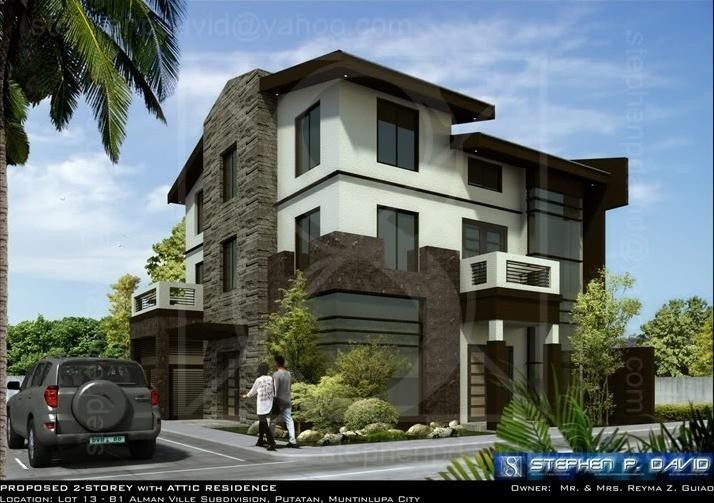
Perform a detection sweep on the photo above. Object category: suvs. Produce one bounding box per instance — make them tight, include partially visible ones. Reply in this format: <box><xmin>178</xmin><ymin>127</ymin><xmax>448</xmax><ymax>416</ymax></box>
<box><xmin>7</xmin><ymin>357</ymin><xmax>162</xmax><ymax>468</ymax></box>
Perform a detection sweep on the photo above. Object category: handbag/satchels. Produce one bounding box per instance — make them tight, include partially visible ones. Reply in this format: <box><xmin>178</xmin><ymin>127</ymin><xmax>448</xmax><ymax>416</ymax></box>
<box><xmin>270</xmin><ymin>396</ymin><xmax>281</xmax><ymax>416</ymax></box>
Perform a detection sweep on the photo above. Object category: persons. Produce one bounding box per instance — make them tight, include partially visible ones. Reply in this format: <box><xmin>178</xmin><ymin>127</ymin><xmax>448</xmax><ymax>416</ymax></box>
<box><xmin>240</xmin><ymin>360</ymin><xmax>277</xmax><ymax>450</ymax></box>
<box><xmin>268</xmin><ymin>355</ymin><xmax>298</xmax><ymax>447</ymax></box>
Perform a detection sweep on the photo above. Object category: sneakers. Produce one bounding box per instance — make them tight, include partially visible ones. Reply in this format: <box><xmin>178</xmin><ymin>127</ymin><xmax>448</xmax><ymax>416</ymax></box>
<box><xmin>253</xmin><ymin>439</ymin><xmax>298</xmax><ymax>451</ymax></box>
